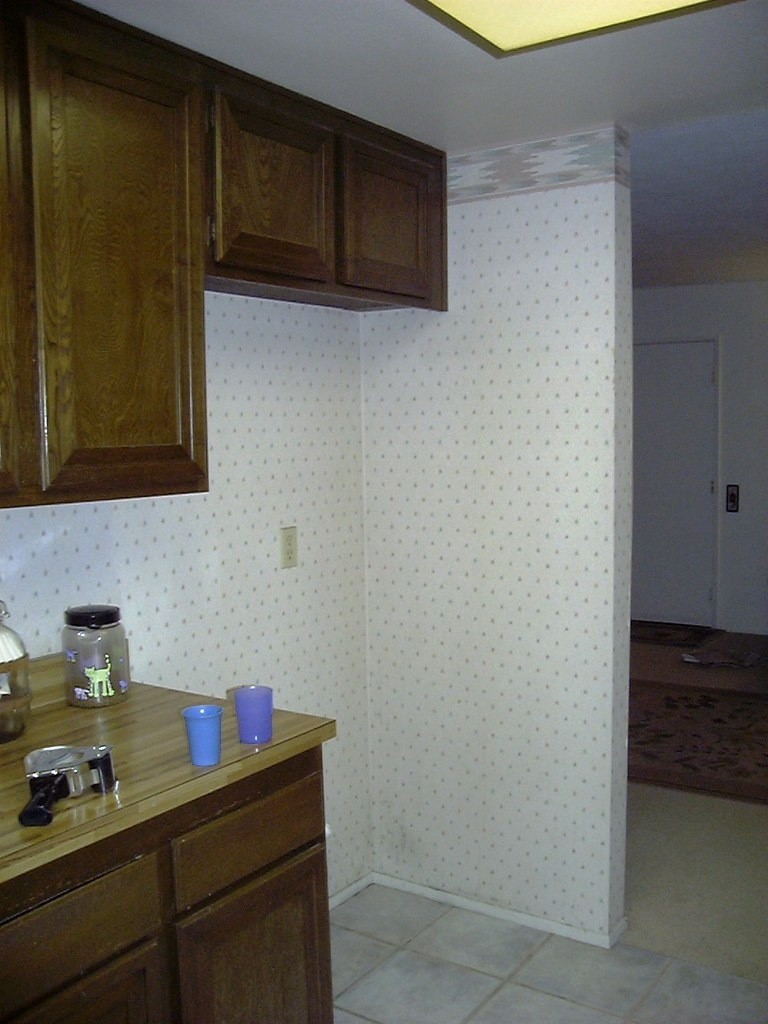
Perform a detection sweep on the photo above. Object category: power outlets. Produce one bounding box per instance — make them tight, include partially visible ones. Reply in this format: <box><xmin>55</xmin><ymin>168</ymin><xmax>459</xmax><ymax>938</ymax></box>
<box><xmin>279</xmin><ymin>526</ymin><xmax>299</xmax><ymax>569</ymax></box>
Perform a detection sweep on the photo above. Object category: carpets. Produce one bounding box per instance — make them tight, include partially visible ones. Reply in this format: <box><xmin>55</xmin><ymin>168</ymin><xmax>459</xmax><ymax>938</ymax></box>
<box><xmin>627</xmin><ymin>678</ymin><xmax>768</xmax><ymax>805</ymax></box>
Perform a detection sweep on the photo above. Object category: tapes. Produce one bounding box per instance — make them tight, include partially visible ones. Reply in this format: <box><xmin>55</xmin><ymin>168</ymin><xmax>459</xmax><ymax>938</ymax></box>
<box><xmin>65</xmin><ymin>761</ymin><xmax>102</xmax><ymax>799</ymax></box>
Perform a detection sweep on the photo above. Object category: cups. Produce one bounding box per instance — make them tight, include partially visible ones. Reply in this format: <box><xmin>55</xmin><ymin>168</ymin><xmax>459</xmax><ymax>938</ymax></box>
<box><xmin>234</xmin><ymin>684</ymin><xmax>273</xmax><ymax>744</ymax></box>
<box><xmin>181</xmin><ymin>705</ymin><xmax>222</xmax><ymax>767</ymax></box>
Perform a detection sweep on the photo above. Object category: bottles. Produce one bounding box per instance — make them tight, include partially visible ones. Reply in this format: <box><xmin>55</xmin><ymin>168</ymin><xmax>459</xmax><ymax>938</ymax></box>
<box><xmin>63</xmin><ymin>605</ymin><xmax>129</xmax><ymax>709</ymax></box>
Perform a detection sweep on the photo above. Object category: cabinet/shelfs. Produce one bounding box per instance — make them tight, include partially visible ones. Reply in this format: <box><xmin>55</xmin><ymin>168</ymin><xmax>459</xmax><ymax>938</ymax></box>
<box><xmin>0</xmin><ymin>654</ymin><xmax>334</xmax><ymax>1024</ymax></box>
<box><xmin>0</xmin><ymin>0</ymin><xmax>447</xmax><ymax>511</ymax></box>
